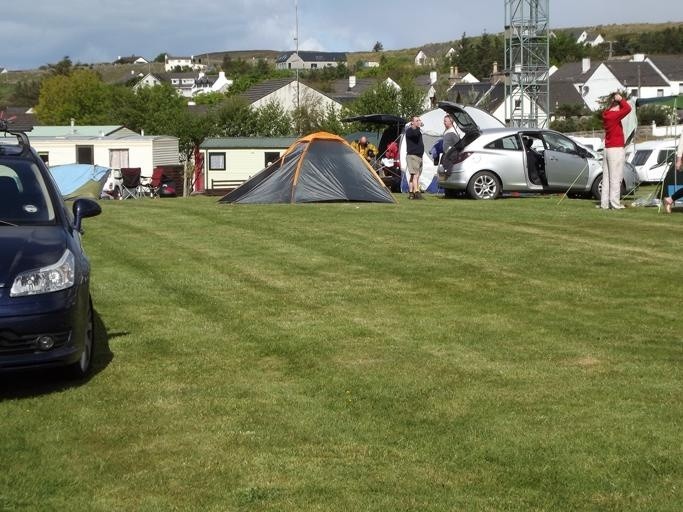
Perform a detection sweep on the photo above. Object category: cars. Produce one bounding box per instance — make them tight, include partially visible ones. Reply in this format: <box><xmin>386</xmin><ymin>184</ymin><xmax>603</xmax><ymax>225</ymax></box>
<box><xmin>433</xmin><ymin>99</ymin><xmax>640</xmax><ymax>201</ymax></box>
<box><xmin>0</xmin><ymin>117</ymin><xmax>104</xmax><ymax>381</ymax></box>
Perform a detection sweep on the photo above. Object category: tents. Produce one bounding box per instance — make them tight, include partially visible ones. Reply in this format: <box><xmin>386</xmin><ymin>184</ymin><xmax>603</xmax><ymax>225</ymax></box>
<box><xmin>48</xmin><ymin>164</ymin><xmax>112</xmax><ymax>200</ymax></box>
<box><xmin>217</xmin><ymin>130</ymin><xmax>399</xmax><ymax>204</ymax></box>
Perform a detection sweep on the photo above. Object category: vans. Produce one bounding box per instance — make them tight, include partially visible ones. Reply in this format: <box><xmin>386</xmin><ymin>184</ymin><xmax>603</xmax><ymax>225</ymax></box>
<box><xmin>102</xmin><ymin>168</ymin><xmax>124</xmax><ymax>197</ymax></box>
<box><xmin>340</xmin><ymin>114</ymin><xmax>409</xmax><ymax>193</ymax></box>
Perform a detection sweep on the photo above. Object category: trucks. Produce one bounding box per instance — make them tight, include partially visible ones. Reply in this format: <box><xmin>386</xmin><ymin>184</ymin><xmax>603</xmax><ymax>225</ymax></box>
<box><xmin>529</xmin><ymin>135</ymin><xmax>678</xmax><ymax>183</ymax></box>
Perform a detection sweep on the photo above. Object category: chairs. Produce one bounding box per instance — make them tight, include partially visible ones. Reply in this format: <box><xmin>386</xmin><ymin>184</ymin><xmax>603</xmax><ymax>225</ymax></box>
<box><xmin>140</xmin><ymin>168</ymin><xmax>165</xmax><ymax>200</ymax></box>
<box><xmin>0</xmin><ymin>175</ymin><xmax>25</xmax><ymax>218</ymax></box>
<box><xmin>120</xmin><ymin>167</ymin><xmax>141</xmax><ymax>200</ymax></box>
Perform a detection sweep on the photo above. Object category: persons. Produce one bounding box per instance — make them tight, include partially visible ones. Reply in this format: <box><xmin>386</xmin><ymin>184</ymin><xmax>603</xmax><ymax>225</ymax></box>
<box><xmin>405</xmin><ymin>115</ymin><xmax>425</xmax><ymax>200</ymax></box>
<box><xmin>350</xmin><ymin>136</ymin><xmax>379</xmax><ymax>163</ymax></box>
<box><xmin>599</xmin><ymin>93</ymin><xmax>632</xmax><ymax>210</ymax></box>
<box><xmin>442</xmin><ymin>115</ymin><xmax>460</xmax><ymax>155</ymax></box>
<box><xmin>663</xmin><ymin>127</ymin><xmax>683</xmax><ymax>214</ymax></box>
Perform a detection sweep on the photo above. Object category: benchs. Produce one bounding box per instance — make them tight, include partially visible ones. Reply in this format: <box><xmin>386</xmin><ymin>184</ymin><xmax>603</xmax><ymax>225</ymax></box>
<box><xmin>205</xmin><ymin>178</ymin><xmax>247</xmax><ymax>197</ymax></box>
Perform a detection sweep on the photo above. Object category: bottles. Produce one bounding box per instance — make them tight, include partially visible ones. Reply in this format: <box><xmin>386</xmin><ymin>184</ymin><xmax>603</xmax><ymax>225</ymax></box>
<box><xmin>420</xmin><ymin>123</ymin><xmax>424</xmax><ymax>127</ymax></box>
<box><xmin>665</xmin><ymin>203</ymin><xmax>671</xmax><ymax>214</ymax></box>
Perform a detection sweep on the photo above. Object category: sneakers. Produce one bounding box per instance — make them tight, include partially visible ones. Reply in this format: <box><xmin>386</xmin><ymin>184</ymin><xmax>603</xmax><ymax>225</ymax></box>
<box><xmin>664</xmin><ymin>197</ymin><xmax>673</xmax><ymax>213</ymax></box>
<box><xmin>410</xmin><ymin>192</ymin><xmax>423</xmax><ymax>200</ymax></box>
<box><xmin>610</xmin><ymin>205</ymin><xmax>625</xmax><ymax>209</ymax></box>
